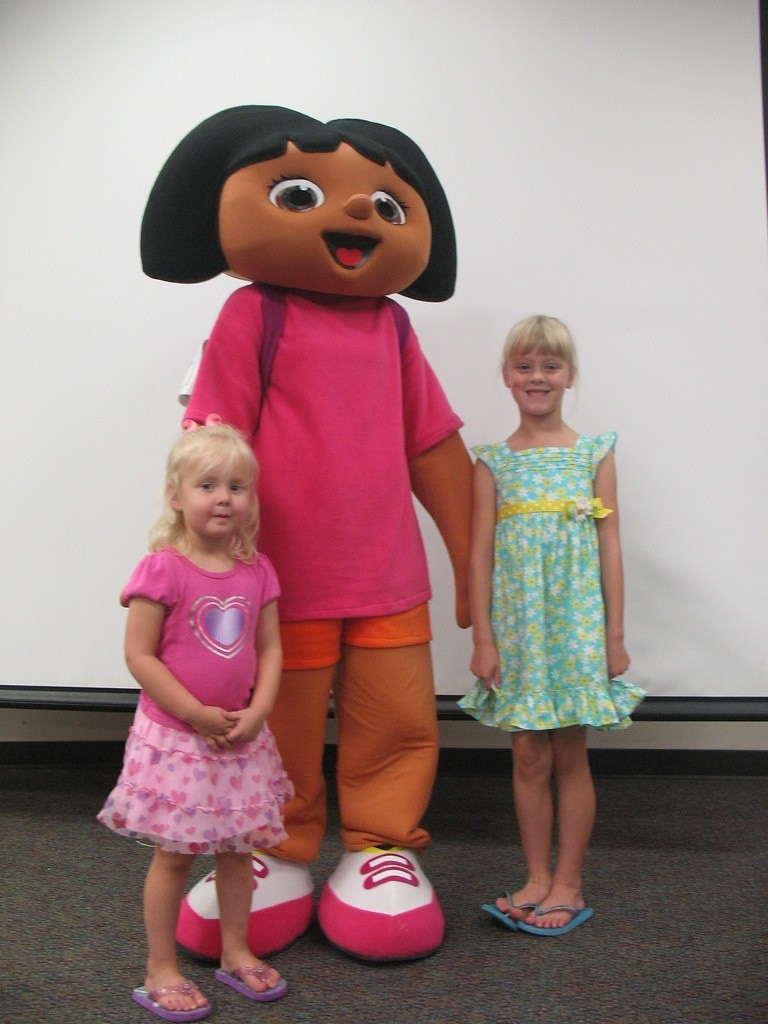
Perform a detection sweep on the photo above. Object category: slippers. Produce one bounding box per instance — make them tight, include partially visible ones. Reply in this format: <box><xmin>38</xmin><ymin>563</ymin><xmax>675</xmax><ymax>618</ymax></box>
<box><xmin>517</xmin><ymin>906</ymin><xmax>594</xmax><ymax>936</ymax></box>
<box><xmin>214</xmin><ymin>962</ymin><xmax>288</xmax><ymax>1002</ymax></box>
<box><xmin>131</xmin><ymin>978</ymin><xmax>212</xmax><ymax>1022</ymax></box>
<box><xmin>480</xmin><ymin>888</ymin><xmax>538</xmax><ymax>932</ymax></box>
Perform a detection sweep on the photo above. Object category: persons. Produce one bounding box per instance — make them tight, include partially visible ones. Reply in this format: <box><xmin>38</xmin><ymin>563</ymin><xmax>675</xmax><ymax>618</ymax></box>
<box><xmin>457</xmin><ymin>315</ymin><xmax>647</xmax><ymax>935</ymax></box>
<box><xmin>97</xmin><ymin>424</ymin><xmax>294</xmax><ymax>1022</ymax></box>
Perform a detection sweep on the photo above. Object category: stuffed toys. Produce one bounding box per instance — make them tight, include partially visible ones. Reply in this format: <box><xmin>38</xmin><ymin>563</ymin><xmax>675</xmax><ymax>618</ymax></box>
<box><xmin>138</xmin><ymin>106</ymin><xmax>475</xmax><ymax>966</ymax></box>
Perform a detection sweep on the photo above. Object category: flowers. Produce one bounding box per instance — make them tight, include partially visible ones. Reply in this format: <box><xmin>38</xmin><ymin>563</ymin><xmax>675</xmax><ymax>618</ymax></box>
<box><xmin>569</xmin><ymin>499</ymin><xmax>593</xmax><ymax>522</ymax></box>
